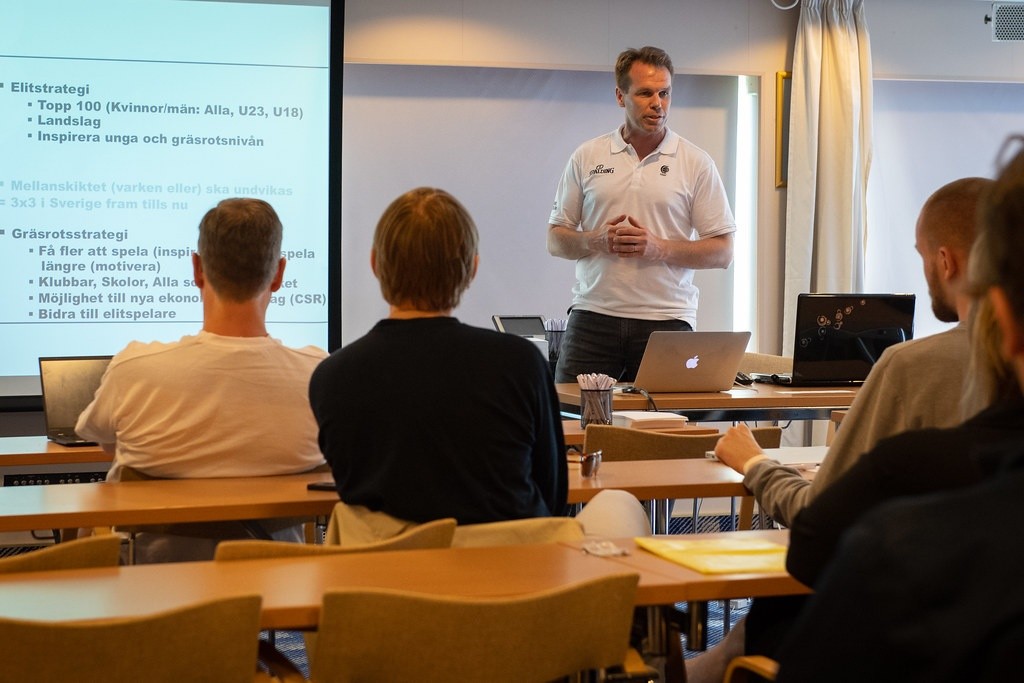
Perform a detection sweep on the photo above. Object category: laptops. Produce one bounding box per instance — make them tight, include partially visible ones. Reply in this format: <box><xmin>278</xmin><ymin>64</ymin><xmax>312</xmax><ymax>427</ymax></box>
<box><xmin>750</xmin><ymin>293</ymin><xmax>916</xmax><ymax>386</ymax></box>
<box><xmin>613</xmin><ymin>332</ymin><xmax>752</xmax><ymax>392</ymax></box>
<box><xmin>38</xmin><ymin>355</ymin><xmax>116</xmax><ymax>447</ymax></box>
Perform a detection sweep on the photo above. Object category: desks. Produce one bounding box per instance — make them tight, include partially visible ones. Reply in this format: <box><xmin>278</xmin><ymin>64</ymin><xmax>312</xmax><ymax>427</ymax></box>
<box><xmin>554</xmin><ymin>378</ymin><xmax>863</xmax><ymax>447</ymax></box>
<box><xmin>0</xmin><ymin>421</ymin><xmax>829</xmax><ymax>683</ymax></box>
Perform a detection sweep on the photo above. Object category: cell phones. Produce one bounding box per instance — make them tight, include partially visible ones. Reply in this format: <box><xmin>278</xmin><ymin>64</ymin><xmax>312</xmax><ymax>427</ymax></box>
<box><xmin>306</xmin><ymin>481</ymin><xmax>337</xmax><ymax>491</ymax></box>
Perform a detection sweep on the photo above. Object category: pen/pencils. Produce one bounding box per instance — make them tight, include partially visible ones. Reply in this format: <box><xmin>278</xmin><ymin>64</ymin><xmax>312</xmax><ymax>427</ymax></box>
<box><xmin>575</xmin><ymin>372</ymin><xmax>618</xmax><ymax>432</ymax></box>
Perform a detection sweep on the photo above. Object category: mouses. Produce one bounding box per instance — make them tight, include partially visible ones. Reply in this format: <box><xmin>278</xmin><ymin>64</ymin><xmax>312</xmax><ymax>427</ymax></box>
<box><xmin>735</xmin><ymin>372</ymin><xmax>753</xmax><ymax>385</ymax></box>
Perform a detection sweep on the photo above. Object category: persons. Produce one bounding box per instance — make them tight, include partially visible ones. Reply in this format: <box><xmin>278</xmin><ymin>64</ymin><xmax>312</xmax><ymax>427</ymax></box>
<box><xmin>683</xmin><ymin>132</ymin><xmax>1024</xmax><ymax>683</ymax></box>
<box><xmin>716</xmin><ymin>178</ymin><xmax>1002</xmax><ymax>526</ymax></box>
<box><xmin>307</xmin><ymin>186</ymin><xmax>570</xmax><ymax>527</ymax></box>
<box><xmin>74</xmin><ymin>198</ymin><xmax>329</xmax><ymax>547</ymax></box>
<box><xmin>545</xmin><ymin>46</ymin><xmax>738</xmax><ymax>423</ymax></box>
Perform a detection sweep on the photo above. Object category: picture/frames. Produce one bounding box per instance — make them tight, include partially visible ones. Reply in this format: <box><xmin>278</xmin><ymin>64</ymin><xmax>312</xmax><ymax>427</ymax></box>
<box><xmin>775</xmin><ymin>72</ymin><xmax>792</xmax><ymax>189</ymax></box>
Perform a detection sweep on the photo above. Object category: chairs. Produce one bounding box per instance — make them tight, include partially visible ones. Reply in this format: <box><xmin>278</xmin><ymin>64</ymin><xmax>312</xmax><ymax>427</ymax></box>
<box><xmin>0</xmin><ymin>533</ymin><xmax>122</xmax><ymax>574</ymax></box>
<box><xmin>583</xmin><ymin>424</ymin><xmax>782</xmax><ymax>535</ymax></box>
<box><xmin>1</xmin><ymin>594</ymin><xmax>272</xmax><ymax>683</ymax></box>
<box><xmin>259</xmin><ymin>571</ymin><xmax>659</xmax><ymax>683</ymax></box>
<box><xmin>739</xmin><ymin>352</ymin><xmax>793</xmax><ymax>427</ymax></box>
<box><xmin>726</xmin><ymin>480</ymin><xmax>1023</xmax><ymax>683</ymax></box>
<box><xmin>213</xmin><ymin>517</ymin><xmax>458</xmax><ymax>649</ymax></box>
<box><xmin>93</xmin><ymin>464</ymin><xmax>332</xmax><ymax>566</ymax></box>
<box><xmin>325</xmin><ymin>488</ymin><xmax>653</xmax><ymax>682</ymax></box>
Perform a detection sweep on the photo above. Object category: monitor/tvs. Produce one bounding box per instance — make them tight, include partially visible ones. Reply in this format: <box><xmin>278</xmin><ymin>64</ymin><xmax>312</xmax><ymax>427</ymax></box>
<box><xmin>492</xmin><ymin>315</ymin><xmax>546</xmax><ymax>340</ymax></box>
